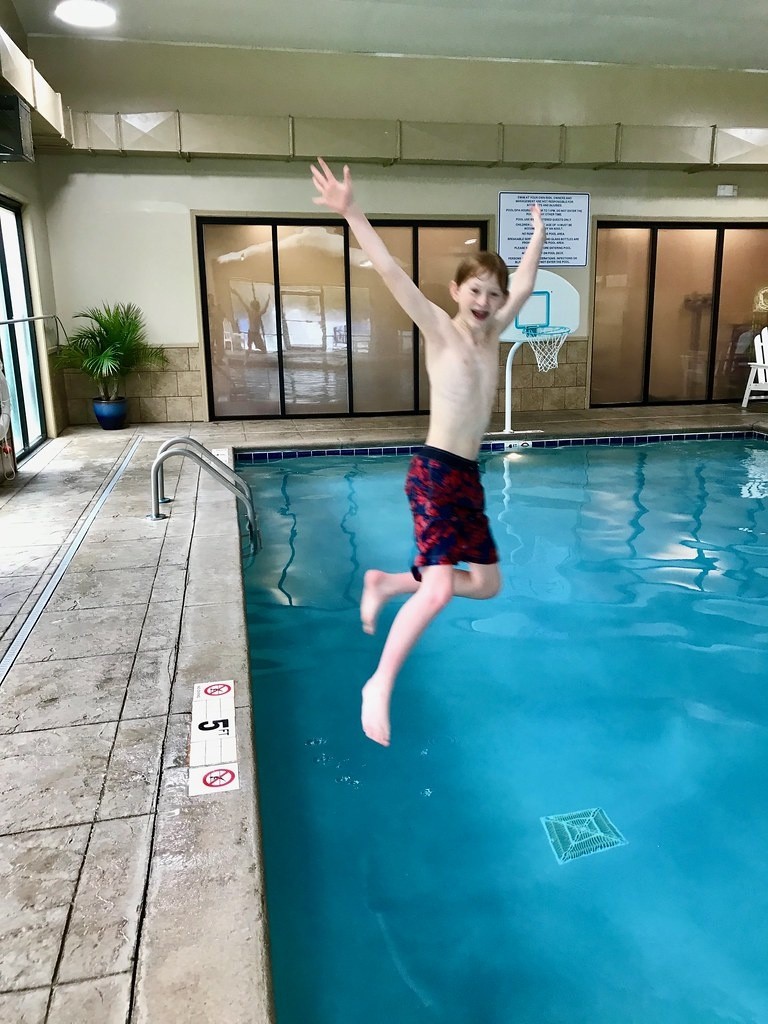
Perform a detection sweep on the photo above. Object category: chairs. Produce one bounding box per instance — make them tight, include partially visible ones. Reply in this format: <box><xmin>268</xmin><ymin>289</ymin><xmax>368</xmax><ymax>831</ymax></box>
<box><xmin>713</xmin><ymin>326</ymin><xmax>752</xmax><ymax>380</ymax></box>
<box><xmin>678</xmin><ymin>334</ymin><xmax>709</xmax><ymax>399</ymax></box>
<box><xmin>741</xmin><ymin>327</ymin><xmax>768</xmax><ymax>407</ymax></box>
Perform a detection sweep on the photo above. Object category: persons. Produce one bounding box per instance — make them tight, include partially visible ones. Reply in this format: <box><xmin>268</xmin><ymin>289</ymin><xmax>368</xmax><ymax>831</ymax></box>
<box><xmin>207</xmin><ymin>293</ymin><xmax>229</xmax><ymax>366</ymax></box>
<box><xmin>309</xmin><ymin>155</ymin><xmax>545</xmax><ymax>747</ymax></box>
<box><xmin>232</xmin><ymin>289</ymin><xmax>271</xmax><ymax>365</ymax></box>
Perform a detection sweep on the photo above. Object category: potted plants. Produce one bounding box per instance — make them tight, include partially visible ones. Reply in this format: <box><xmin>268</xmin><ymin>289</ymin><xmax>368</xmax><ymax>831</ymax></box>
<box><xmin>52</xmin><ymin>298</ymin><xmax>172</xmax><ymax>431</ymax></box>
